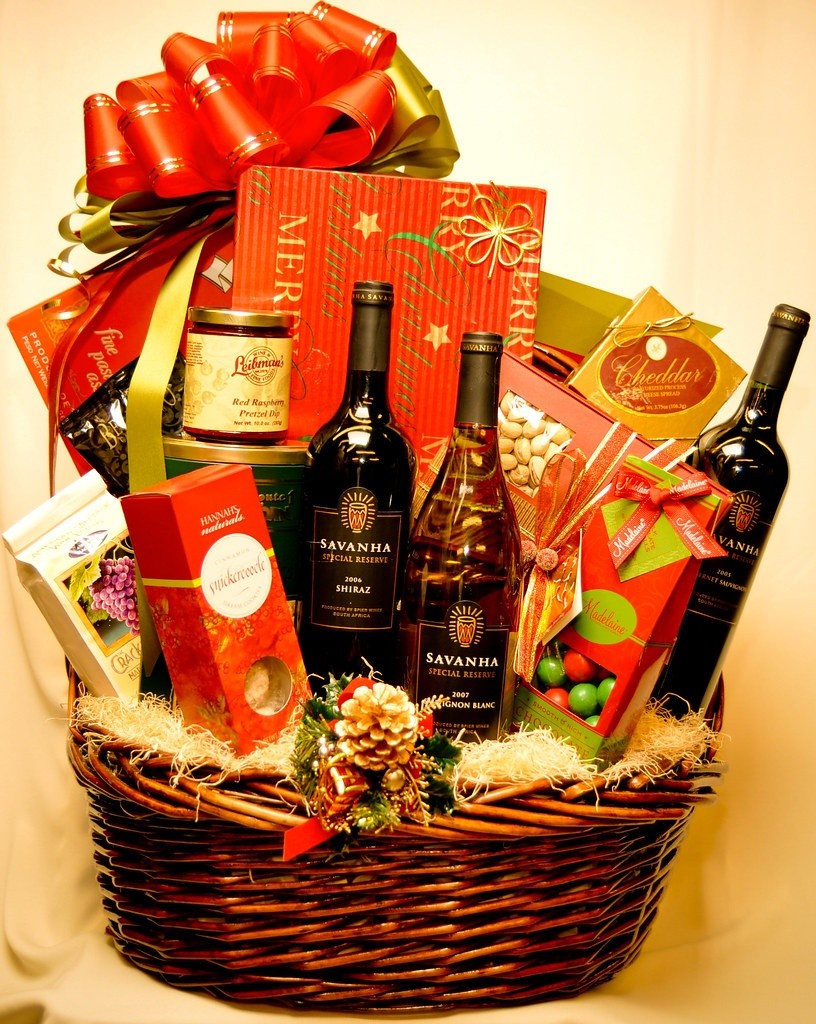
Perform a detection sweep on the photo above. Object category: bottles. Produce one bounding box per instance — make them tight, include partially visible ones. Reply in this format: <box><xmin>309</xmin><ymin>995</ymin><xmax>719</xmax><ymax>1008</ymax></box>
<box><xmin>643</xmin><ymin>303</ymin><xmax>811</xmax><ymax>728</ymax></box>
<box><xmin>395</xmin><ymin>331</ymin><xmax>525</xmax><ymax>744</ymax></box>
<box><xmin>297</xmin><ymin>279</ymin><xmax>407</xmax><ymax>699</ymax></box>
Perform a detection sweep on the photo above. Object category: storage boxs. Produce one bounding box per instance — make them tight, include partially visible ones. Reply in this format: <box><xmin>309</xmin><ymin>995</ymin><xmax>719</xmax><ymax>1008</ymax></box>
<box><xmin>0</xmin><ymin>166</ymin><xmax>735</xmax><ymax>772</ymax></box>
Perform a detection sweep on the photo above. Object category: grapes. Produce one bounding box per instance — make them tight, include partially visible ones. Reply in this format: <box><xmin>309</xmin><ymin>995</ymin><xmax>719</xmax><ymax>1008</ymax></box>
<box><xmin>88</xmin><ymin>548</ymin><xmax>141</xmax><ymax>636</ymax></box>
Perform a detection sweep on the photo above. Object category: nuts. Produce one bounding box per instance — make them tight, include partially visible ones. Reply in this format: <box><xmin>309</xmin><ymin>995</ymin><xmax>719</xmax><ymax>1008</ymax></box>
<box><xmin>494</xmin><ymin>390</ymin><xmax>575</xmax><ymax>499</ymax></box>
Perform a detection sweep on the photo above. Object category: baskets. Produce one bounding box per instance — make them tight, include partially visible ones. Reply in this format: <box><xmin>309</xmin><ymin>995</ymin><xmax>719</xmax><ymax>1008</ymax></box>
<box><xmin>64</xmin><ymin>658</ymin><xmax>725</xmax><ymax>1011</ymax></box>
